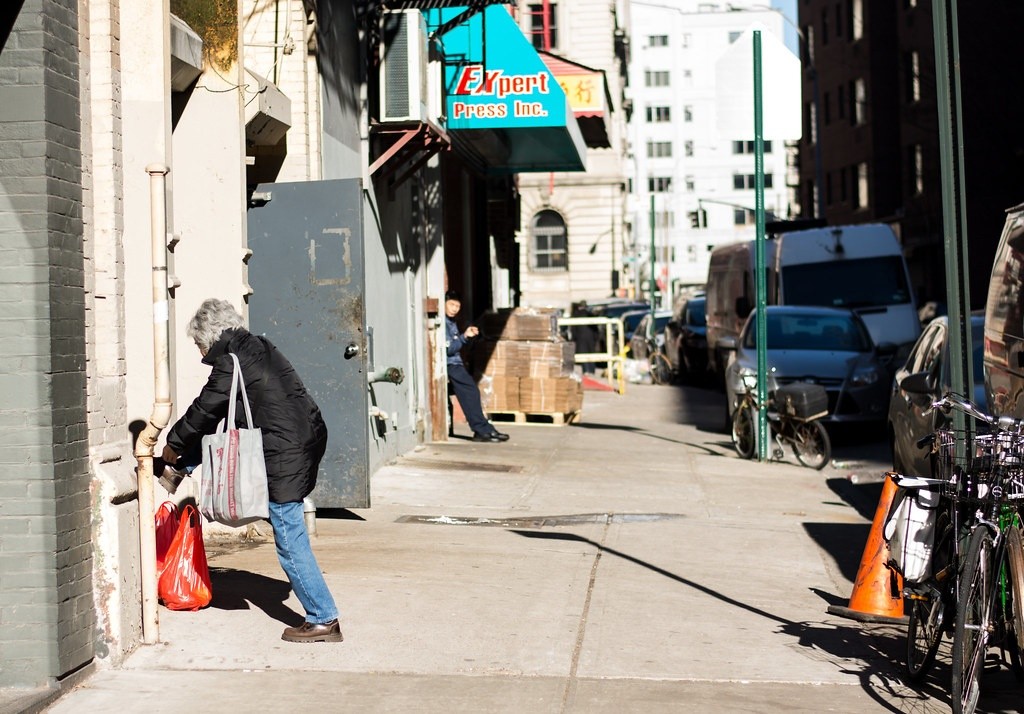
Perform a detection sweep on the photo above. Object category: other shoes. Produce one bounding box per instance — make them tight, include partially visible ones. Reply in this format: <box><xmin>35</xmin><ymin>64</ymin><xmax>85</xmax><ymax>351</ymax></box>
<box><xmin>472</xmin><ymin>430</ymin><xmax>510</xmax><ymax>443</ymax></box>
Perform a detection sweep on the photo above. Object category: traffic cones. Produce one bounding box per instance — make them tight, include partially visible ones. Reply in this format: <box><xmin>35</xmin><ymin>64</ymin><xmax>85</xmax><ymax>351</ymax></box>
<box><xmin>826</xmin><ymin>471</ymin><xmax>911</xmax><ymax>627</ymax></box>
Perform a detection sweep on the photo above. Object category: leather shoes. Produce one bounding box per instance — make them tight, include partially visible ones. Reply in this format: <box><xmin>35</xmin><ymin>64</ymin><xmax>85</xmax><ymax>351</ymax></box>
<box><xmin>281</xmin><ymin>618</ymin><xmax>343</xmax><ymax>642</ymax></box>
<box><xmin>152</xmin><ymin>456</ymin><xmax>192</xmax><ymax>495</ymax></box>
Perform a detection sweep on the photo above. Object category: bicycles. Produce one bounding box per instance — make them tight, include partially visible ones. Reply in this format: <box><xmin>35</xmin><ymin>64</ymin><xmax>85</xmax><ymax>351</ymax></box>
<box><xmin>888</xmin><ymin>388</ymin><xmax>1024</xmax><ymax>714</ymax></box>
<box><xmin>645</xmin><ymin>335</ymin><xmax>671</xmax><ymax>386</ymax></box>
<box><xmin>727</xmin><ymin>368</ymin><xmax>832</xmax><ymax>471</ymax></box>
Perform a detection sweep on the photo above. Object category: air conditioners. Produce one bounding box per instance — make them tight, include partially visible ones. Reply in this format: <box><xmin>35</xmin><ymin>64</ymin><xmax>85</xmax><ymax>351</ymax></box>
<box><xmin>379</xmin><ymin>9</ymin><xmax>446</xmax><ymax>137</ymax></box>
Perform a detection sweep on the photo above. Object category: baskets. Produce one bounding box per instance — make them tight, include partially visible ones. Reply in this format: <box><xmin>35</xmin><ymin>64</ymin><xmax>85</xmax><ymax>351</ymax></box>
<box><xmin>935</xmin><ymin>427</ymin><xmax>1024</xmax><ymax>506</ymax></box>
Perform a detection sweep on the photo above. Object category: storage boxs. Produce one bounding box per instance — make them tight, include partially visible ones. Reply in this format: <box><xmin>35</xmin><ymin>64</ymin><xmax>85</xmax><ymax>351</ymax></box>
<box><xmin>464</xmin><ymin>308</ymin><xmax>584</xmax><ymax>414</ymax></box>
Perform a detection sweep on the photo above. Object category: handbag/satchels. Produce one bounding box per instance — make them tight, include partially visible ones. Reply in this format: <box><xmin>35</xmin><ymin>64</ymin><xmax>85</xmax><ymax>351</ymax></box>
<box><xmin>155</xmin><ymin>501</ymin><xmax>213</xmax><ymax>611</ymax></box>
<box><xmin>201</xmin><ymin>352</ymin><xmax>269</xmax><ymax>527</ymax></box>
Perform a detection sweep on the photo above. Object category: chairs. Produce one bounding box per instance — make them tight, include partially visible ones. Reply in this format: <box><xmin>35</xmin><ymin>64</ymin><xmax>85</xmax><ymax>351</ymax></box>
<box><xmin>766</xmin><ymin>318</ymin><xmax>847</xmax><ymax>348</ymax></box>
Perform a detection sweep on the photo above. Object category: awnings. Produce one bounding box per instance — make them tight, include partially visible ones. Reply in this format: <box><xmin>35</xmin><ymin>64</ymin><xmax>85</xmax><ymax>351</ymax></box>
<box><xmin>532</xmin><ymin>45</ymin><xmax>615</xmax><ymax>148</ymax></box>
<box><xmin>423</xmin><ymin>1</ymin><xmax>590</xmax><ymax>173</ymax></box>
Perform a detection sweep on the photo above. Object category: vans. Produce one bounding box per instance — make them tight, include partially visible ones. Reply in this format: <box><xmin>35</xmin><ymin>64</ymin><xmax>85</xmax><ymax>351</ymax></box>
<box><xmin>774</xmin><ymin>222</ymin><xmax>920</xmax><ymax>378</ymax></box>
<box><xmin>704</xmin><ymin>239</ymin><xmax>777</xmax><ymax>375</ymax></box>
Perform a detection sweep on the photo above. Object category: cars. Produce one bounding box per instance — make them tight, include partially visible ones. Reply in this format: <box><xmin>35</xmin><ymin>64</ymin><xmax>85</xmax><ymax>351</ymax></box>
<box><xmin>715</xmin><ymin>303</ymin><xmax>885</xmax><ymax>445</ymax></box>
<box><xmin>664</xmin><ymin>290</ymin><xmax>706</xmax><ymax>385</ymax></box>
<box><xmin>887</xmin><ymin>307</ymin><xmax>993</xmax><ymax>481</ymax></box>
<box><xmin>615</xmin><ymin>311</ymin><xmax>647</xmax><ymax>361</ymax></box>
<box><xmin>628</xmin><ymin>312</ymin><xmax>673</xmax><ymax>362</ymax></box>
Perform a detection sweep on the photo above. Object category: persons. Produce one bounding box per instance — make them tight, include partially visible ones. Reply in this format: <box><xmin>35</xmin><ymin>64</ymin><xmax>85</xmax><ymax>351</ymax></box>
<box><xmin>571</xmin><ymin>300</ymin><xmax>600</xmax><ymax>378</ymax></box>
<box><xmin>446</xmin><ymin>289</ymin><xmax>510</xmax><ymax>442</ymax></box>
<box><xmin>154</xmin><ymin>299</ymin><xmax>345</xmax><ymax>642</ymax></box>
